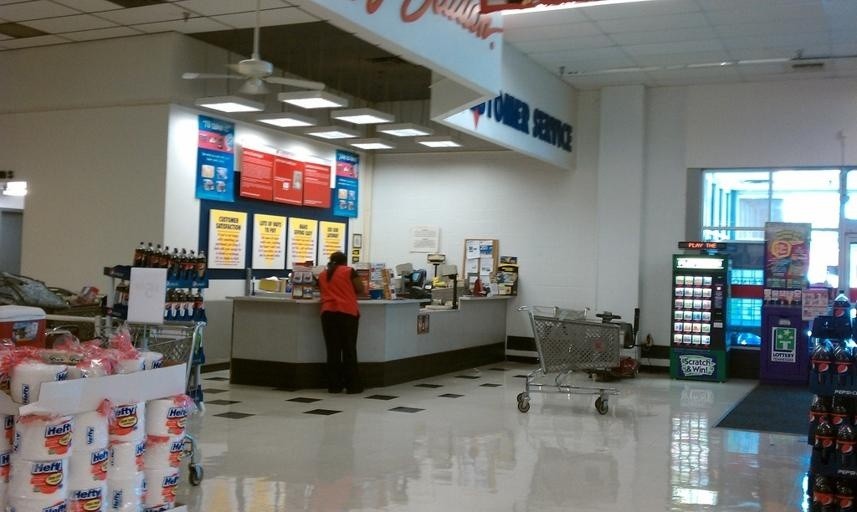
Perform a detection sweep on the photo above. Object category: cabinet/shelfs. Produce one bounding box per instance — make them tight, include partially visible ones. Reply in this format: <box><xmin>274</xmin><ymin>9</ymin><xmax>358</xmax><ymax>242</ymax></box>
<box><xmin>98</xmin><ymin>261</ymin><xmax>206</xmax><ymax>417</ymax></box>
<box><xmin>804</xmin><ymin>308</ymin><xmax>857</xmax><ymax>512</ymax></box>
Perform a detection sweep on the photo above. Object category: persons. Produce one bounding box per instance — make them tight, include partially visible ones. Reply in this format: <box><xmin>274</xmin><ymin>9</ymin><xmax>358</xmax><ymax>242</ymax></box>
<box><xmin>317</xmin><ymin>249</ymin><xmax>367</xmax><ymax>396</ymax></box>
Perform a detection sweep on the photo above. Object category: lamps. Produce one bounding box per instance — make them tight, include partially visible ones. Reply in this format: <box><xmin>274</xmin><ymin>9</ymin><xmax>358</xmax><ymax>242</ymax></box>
<box><xmin>193</xmin><ymin>25</ymin><xmax>462</xmax><ymax>155</ymax></box>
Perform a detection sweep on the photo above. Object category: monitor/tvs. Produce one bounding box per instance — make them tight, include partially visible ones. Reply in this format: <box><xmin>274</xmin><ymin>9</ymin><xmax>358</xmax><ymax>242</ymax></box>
<box><xmin>412</xmin><ymin>270</ymin><xmax>427</xmax><ymax>286</ymax></box>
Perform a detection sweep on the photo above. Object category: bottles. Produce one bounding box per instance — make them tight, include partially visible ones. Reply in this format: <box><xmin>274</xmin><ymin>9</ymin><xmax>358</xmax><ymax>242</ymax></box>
<box><xmin>113</xmin><ymin>241</ymin><xmax>208</xmax><ymax>323</ymax></box>
<box><xmin>807</xmin><ymin>290</ymin><xmax>856</xmax><ymax>512</ymax></box>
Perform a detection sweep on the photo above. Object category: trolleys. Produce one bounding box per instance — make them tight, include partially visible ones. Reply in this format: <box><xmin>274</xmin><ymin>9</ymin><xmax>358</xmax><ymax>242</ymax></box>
<box><xmin>516</xmin><ymin>304</ymin><xmax>619</xmax><ymax>414</ymax></box>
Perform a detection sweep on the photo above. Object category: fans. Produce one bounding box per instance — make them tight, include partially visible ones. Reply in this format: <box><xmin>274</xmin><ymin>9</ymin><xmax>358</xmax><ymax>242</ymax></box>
<box><xmin>183</xmin><ymin>1</ymin><xmax>325</xmax><ymax>96</ymax></box>
